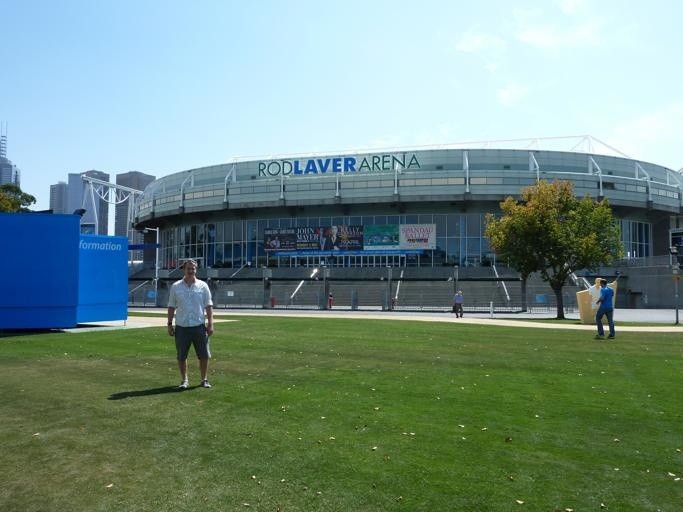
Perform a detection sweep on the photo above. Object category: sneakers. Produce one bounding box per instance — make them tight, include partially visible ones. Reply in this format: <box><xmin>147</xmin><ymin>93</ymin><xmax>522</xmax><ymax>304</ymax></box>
<box><xmin>608</xmin><ymin>335</ymin><xmax>615</xmax><ymax>339</ymax></box>
<box><xmin>179</xmin><ymin>380</ymin><xmax>189</xmax><ymax>389</ymax></box>
<box><xmin>592</xmin><ymin>334</ymin><xmax>604</xmax><ymax>339</ymax></box>
<box><xmin>200</xmin><ymin>379</ymin><xmax>212</xmax><ymax>388</ymax></box>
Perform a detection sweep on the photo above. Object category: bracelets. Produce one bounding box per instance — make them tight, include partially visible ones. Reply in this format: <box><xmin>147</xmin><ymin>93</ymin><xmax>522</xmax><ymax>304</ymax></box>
<box><xmin>167</xmin><ymin>322</ymin><xmax>172</xmax><ymax>326</ymax></box>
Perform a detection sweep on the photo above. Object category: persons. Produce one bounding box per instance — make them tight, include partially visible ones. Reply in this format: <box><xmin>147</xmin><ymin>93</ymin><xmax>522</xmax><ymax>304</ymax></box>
<box><xmin>166</xmin><ymin>258</ymin><xmax>213</xmax><ymax>389</ymax></box>
<box><xmin>320</xmin><ymin>226</ymin><xmax>326</xmax><ymax>250</ymax></box>
<box><xmin>594</xmin><ymin>279</ymin><xmax>617</xmax><ymax>339</ymax></box>
<box><xmin>451</xmin><ymin>291</ymin><xmax>464</xmax><ymax>318</ymax></box>
<box><xmin>269</xmin><ymin>236</ymin><xmax>280</xmax><ymax>247</ymax></box>
<box><xmin>323</xmin><ymin>225</ymin><xmax>341</xmax><ymax>251</ymax></box>
